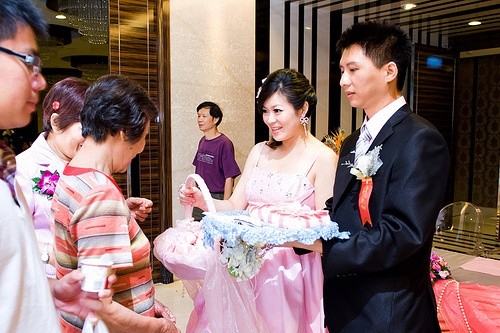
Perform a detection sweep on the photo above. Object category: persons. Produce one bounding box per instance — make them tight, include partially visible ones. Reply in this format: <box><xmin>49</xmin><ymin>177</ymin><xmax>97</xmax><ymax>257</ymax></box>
<box><xmin>179</xmin><ymin>68</ymin><xmax>338</xmax><ymax>333</ymax></box>
<box><xmin>282</xmin><ymin>20</ymin><xmax>450</xmax><ymax>333</ymax></box>
<box><xmin>0</xmin><ymin>0</ymin><xmax>117</xmax><ymax>333</ymax></box>
<box><xmin>14</xmin><ymin>76</ymin><xmax>153</xmax><ymax>282</ymax></box>
<box><xmin>190</xmin><ymin>101</ymin><xmax>242</xmax><ymax>223</ymax></box>
<box><xmin>51</xmin><ymin>74</ymin><xmax>181</xmax><ymax>333</ymax></box>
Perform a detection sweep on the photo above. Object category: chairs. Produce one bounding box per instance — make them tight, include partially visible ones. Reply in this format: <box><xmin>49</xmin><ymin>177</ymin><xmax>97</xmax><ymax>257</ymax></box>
<box><xmin>434</xmin><ymin>200</ymin><xmax>490</xmax><ymax>259</ymax></box>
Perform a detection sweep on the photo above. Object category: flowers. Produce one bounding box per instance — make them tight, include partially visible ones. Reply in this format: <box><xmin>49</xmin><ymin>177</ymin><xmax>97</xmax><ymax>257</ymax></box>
<box><xmin>341</xmin><ymin>144</ymin><xmax>383</xmax><ymax>180</ymax></box>
<box><xmin>30</xmin><ymin>169</ymin><xmax>59</xmax><ymax>200</ymax></box>
<box><xmin>429</xmin><ymin>249</ymin><xmax>451</xmax><ymax>282</ymax></box>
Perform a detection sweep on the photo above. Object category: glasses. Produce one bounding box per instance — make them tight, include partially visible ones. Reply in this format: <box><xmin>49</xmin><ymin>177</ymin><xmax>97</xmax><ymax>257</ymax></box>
<box><xmin>0</xmin><ymin>46</ymin><xmax>43</xmax><ymax>76</ymax></box>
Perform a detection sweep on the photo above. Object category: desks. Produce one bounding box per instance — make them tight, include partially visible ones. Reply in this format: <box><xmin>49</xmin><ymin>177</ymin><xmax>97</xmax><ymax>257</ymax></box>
<box><xmin>432</xmin><ymin>246</ymin><xmax>500</xmax><ymax>286</ymax></box>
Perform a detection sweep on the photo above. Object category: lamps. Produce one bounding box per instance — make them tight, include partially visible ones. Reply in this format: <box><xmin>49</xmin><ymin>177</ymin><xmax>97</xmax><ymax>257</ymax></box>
<box><xmin>46</xmin><ymin>0</ymin><xmax>109</xmax><ymax>44</ymax></box>
<box><xmin>71</xmin><ymin>57</ymin><xmax>108</xmax><ymax>82</ymax></box>
<box><xmin>40</xmin><ymin>24</ymin><xmax>72</xmax><ymax>65</ymax></box>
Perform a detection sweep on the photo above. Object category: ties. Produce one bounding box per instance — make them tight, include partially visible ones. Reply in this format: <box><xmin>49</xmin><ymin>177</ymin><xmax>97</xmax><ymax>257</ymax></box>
<box><xmin>354</xmin><ymin>124</ymin><xmax>372</xmax><ymax>167</ymax></box>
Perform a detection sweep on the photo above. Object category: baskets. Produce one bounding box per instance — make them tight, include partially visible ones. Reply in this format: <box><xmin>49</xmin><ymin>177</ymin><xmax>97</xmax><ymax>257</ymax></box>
<box><xmin>153</xmin><ymin>174</ymin><xmax>216</xmax><ymax>280</ymax></box>
